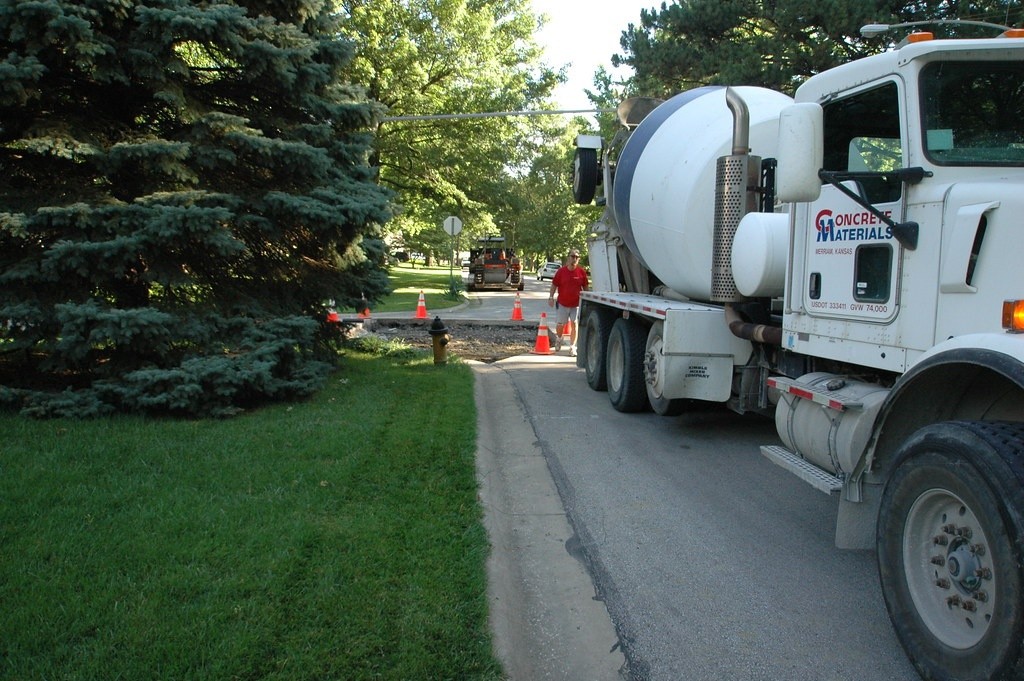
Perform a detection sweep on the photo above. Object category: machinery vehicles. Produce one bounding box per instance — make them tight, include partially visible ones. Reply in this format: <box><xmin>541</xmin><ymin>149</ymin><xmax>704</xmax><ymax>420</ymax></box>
<box><xmin>568</xmin><ymin>17</ymin><xmax>1023</xmax><ymax>681</ymax></box>
<box><xmin>468</xmin><ymin>230</ymin><xmax>524</xmax><ymax>291</ymax></box>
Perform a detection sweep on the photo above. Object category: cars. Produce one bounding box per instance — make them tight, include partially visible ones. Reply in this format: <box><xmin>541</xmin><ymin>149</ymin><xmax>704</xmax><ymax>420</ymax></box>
<box><xmin>536</xmin><ymin>262</ymin><xmax>563</xmax><ymax>281</ymax></box>
<box><xmin>390</xmin><ymin>252</ymin><xmax>409</xmax><ymax>262</ymax></box>
<box><xmin>461</xmin><ymin>258</ymin><xmax>470</xmax><ymax>269</ymax></box>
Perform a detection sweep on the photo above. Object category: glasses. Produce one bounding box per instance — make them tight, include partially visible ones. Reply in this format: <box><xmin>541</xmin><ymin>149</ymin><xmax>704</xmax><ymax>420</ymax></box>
<box><xmin>569</xmin><ymin>255</ymin><xmax>580</xmax><ymax>258</ymax></box>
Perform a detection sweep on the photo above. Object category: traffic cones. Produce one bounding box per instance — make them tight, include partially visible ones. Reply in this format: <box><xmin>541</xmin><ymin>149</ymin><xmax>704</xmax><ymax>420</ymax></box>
<box><xmin>510</xmin><ymin>292</ymin><xmax>525</xmax><ymax>321</ymax></box>
<box><xmin>562</xmin><ymin>316</ymin><xmax>572</xmax><ymax>336</ymax></box>
<box><xmin>415</xmin><ymin>291</ymin><xmax>429</xmax><ymax>318</ymax></box>
<box><xmin>326</xmin><ymin>299</ymin><xmax>344</xmax><ymax>332</ymax></box>
<box><xmin>357</xmin><ymin>292</ymin><xmax>372</xmax><ymax>320</ymax></box>
<box><xmin>529</xmin><ymin>313</ymin><xmax>555</xmax><ymax>355</ymax></box>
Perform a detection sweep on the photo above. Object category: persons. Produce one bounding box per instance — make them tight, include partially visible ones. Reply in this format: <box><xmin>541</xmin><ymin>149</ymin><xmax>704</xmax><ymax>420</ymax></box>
<box><xmin>548</xmin><ymin>247</ymin><xmax>590</xmax><ymax>358</ymax></box>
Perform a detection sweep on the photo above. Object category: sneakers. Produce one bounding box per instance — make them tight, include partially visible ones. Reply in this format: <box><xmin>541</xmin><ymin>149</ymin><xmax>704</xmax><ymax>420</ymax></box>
<box><xmin>569</xmin><ymin>348</ymin><xmax>577</xmax><ymax>357</ymax></box>
<box><xmin>555</xmin><ymin>337</ymin><xmax>562</xmax><ymax>351</ymax></box>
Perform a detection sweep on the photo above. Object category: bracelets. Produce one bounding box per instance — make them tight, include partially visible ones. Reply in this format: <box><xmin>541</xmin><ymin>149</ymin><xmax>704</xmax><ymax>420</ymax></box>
<box><xmin>549</xmin><ymin>296</ymin><xmax>553</xmax><ymax>301</ymax></box>
